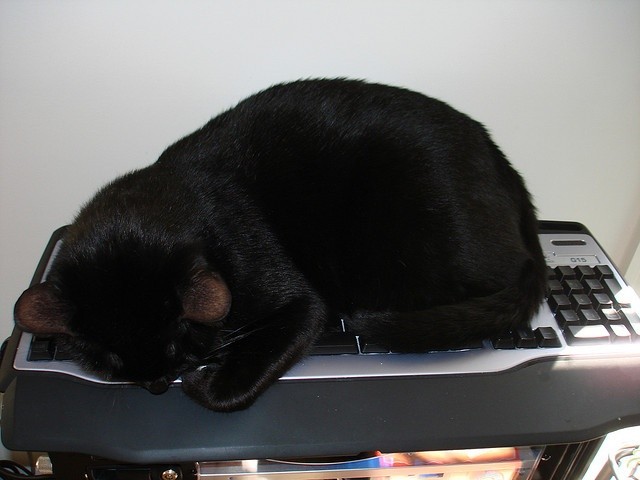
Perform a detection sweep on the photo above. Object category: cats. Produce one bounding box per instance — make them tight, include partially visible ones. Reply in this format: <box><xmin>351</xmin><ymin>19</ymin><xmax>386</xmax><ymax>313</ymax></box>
<box><xmin>13</xmin><ymin>76</ymin><xmax>546</xmax><ymax>413</ymax></box>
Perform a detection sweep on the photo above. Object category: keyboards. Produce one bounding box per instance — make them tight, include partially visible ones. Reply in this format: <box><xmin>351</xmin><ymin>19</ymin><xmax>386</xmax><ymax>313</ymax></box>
<box><xmin>0</xmin><ymin>221</ymin><xmax>640</xmax><ymax>464</ymax></box>
